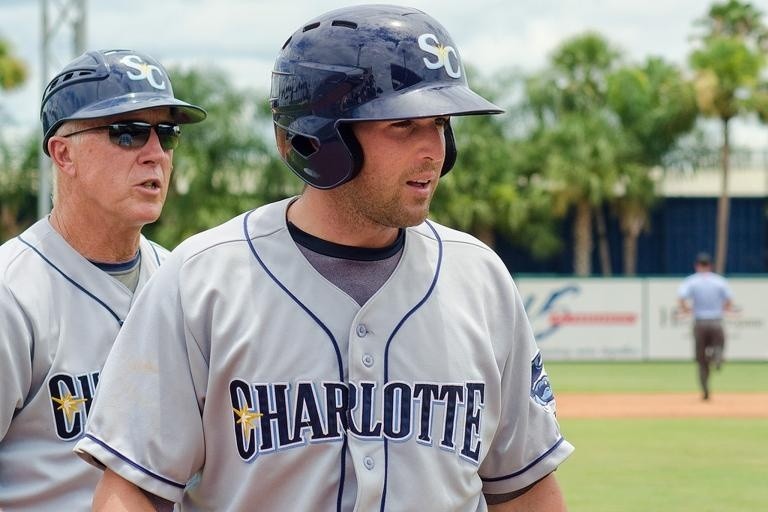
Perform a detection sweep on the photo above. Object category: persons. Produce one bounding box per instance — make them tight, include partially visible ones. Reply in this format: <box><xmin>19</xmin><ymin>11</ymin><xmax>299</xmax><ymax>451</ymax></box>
<box><xmin>0</xmin><ymin>48</ymin><xmax>209</xmax><ymax>512</ymax></box>
<box><xmin>677</xmin><ymin>253</ymin><xmax>732</xmax><ymax>401</ymax></box>
<box><xmin>74</xmin><ymin>4</ymin><xmax>576</xmax><ymax>511</ymax></box>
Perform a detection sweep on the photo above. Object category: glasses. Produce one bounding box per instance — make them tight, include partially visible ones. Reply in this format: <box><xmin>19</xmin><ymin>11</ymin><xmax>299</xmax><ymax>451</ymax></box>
<box><xmin>60</xmin><ymin>119</ymin><xmax>181</xmax><ymax>153</ymax></box>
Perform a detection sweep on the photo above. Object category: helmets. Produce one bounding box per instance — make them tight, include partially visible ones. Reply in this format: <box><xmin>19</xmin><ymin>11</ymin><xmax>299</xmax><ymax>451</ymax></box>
<box><xmin>269</xmin><ymin>4</ymin><xmax>506</xmax><ymax>190</ymax></box>
<box><xmin>39</xmin><ymin>48</ymin><xmax>207</xmax><ymax>157</ymax></box>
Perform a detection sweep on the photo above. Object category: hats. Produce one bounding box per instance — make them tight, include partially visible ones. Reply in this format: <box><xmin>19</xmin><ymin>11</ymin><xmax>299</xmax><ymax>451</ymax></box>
<box><xmin>692</xmin><ymin>252</ymin><xmax>711</xmax><ymax>264</ymax></box>
